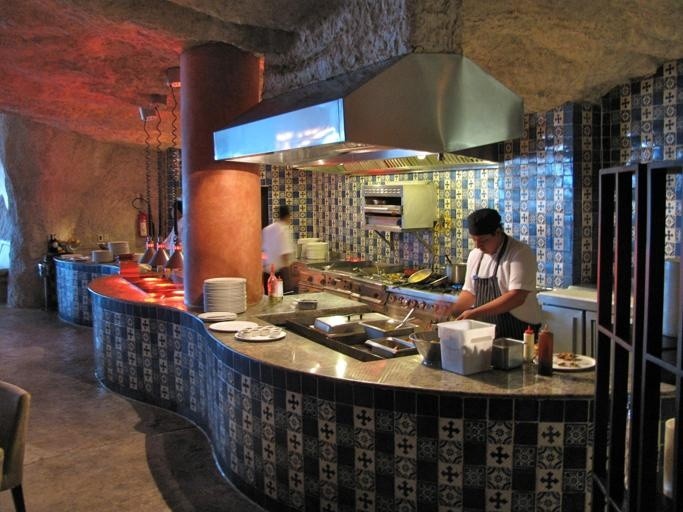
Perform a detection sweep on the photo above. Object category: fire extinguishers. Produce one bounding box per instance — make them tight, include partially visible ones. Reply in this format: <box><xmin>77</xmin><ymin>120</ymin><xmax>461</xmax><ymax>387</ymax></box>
<box><xmin>131</xmin><ymin>197</ymin><xmax>149</xmax><ymax>238</ymax></box>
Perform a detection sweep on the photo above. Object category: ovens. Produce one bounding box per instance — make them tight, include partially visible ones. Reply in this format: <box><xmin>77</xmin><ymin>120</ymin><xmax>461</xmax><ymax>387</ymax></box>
<box><xmin>360</xmin><ymin>184</ymin><xmax>438</xmax><ymax>233</ymax></box>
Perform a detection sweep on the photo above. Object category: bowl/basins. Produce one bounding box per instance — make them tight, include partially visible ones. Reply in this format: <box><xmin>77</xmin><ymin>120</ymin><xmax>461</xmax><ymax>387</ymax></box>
<box><xmin>492</xmin><ymin>338</ymin><xmax>524</xmax><ymax>370</ymax></box>
<box><xmin>358</xmin><ymin>319</ymin><xmax>417</xmax><ymax>338</ymax></box>
<box><xmin>409</xmin><ymin>331</ymin><xmax>440</xmax><ymax>366</ymax></box>
<box><xmin>364</xmin><ymin>336</ymin><xmax>417</xmax><ymax>360</ymax></box>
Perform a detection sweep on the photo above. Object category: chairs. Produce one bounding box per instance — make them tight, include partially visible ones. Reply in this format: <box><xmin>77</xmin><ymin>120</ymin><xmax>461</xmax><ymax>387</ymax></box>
<box><xmin>0</xmin><ymin>381</ymin><xmax>31</xmax><ymax>512</ymax></box>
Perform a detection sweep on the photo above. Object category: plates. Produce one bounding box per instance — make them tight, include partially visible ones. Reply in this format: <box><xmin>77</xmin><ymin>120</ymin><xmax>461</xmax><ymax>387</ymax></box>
<box><xmin>197</xmin><ymin>312</ymin><xmax>237</xmax><ymax>322</ymax></box>
<box><xmin>108</xmin><ymin>241</ymin><xmax>129</xmax><ymax>257</ymax></box>
<box><xmin>208</xmin><ymin>320</ymin><xmax>258</xmax><ymax>332</ymax></box>
<box><xmin>92</xmin><ymin>250</ymin><xmax>113</xmax><ymax>263</ymax></box>
<box><xmin>297</xmin><ymin>237</ymin><xmax>329</xmax><ymax>260</ymax></box>
<box><xmin>532</xmin><ymin>353</ymin><xmax>596</xmax><ymax>371</ymax></box>
<box><xmin>203</xmin><ymin>277</ymin><xmax>246</xmax><ymax>315</ymax></box>
<box><xmin>234</xmin><ymin>326</ymin><xmax>288</xmax><ymax>343</ymax></box>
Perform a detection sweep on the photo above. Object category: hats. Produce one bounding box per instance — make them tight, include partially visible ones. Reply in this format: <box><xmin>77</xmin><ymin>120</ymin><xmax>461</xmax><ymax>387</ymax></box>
<box><xmin>468</xmin><ymin>208</ymin><xmax>501</xmax><ymax>236</ymax></box>
<box><xmin>169</xmin><ymin>201</ymin><xmax>183</xmax><ymax>210</ymax></box>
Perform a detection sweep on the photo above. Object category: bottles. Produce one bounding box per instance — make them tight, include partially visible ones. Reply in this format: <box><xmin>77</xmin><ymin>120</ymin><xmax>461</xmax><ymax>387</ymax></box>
<box><xmin>268</xmin><ymin>263</ymin><xmax>277</xmax><ymax>306</ymax></box>
<box><xmin>52</xmin><ymin>234</ymin><xmax>58</xmax><ymax>252</ymax></box>
<box><xmin>537</xmin><ymin>323</ymin><xmax>553</xmax><ymax>375</ymax></box>
<box><xmin>277</xmin><ymin>276</ymin><xmax>284</xmax><ymax>302</ymax></box>
<box><xmin>524</xmin><ymin>325</ymin><xmax>535</xmax><ymax>361</ymax></box>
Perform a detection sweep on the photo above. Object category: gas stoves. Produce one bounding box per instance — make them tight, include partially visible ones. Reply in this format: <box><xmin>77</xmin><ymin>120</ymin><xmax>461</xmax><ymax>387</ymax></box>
<box><xmin>385</xmin><ymin>276</ymin><xmax>476</xmax><ymax>315</ymax></box>
<box><xmin>350</xmin><ymin>272</ymin><xmax>406</xmax><ymax>314</ymax></box>
<box><xmin>325</xmin><ymin>266</ymin><xmax>373</xmax><ymax>298</ymax></box>
<box><xmin>297</xmin><ymin>260</ymin><xmax>347</xmax><ymax>294</ymax></box>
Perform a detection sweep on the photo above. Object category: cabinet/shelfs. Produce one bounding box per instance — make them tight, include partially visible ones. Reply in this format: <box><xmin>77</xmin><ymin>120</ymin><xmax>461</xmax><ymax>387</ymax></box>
<box><xmin>539</xmin><ymin>303</ymin><xmax>633</xmax><ymax>371</ymax></box>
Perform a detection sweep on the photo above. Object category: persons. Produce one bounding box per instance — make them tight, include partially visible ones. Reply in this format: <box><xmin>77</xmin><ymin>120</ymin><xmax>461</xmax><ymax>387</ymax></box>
<box><xmin>157</xmin><ymin>200</ymin><xmax>185</xmax><ymax>255</ymax></box>
<box><xmin>262</xmin><ymin>205</ymin><xmax>301</xmax><ymax>294</ymax></box>
<box><xmin>450</xmin><ymin>206</ymin><xmax>543</xmax><ymax>340</ymax></box>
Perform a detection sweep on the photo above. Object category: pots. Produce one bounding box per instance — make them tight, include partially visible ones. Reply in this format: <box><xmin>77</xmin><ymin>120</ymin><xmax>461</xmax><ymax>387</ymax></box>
<box><xmin>346</xmin><ymin>255</ymin><xmax>370</xmax><ymax>268</ymax></box>
<box><xmin>446</xmin><ymin>263</ymin><xmax>467</xmax><ymax>284</ymax></box>
<box><xmin>391</xmin><ymin>268</ymin><xmax>433</xmax><ymax>290</ymax></box>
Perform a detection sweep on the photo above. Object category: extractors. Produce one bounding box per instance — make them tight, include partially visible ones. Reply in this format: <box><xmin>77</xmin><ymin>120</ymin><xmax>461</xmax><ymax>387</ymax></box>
<box><xmin>212</xmin><ymin>52</ymin><xmax>526</xmax><ymax>175</ymax></box>
